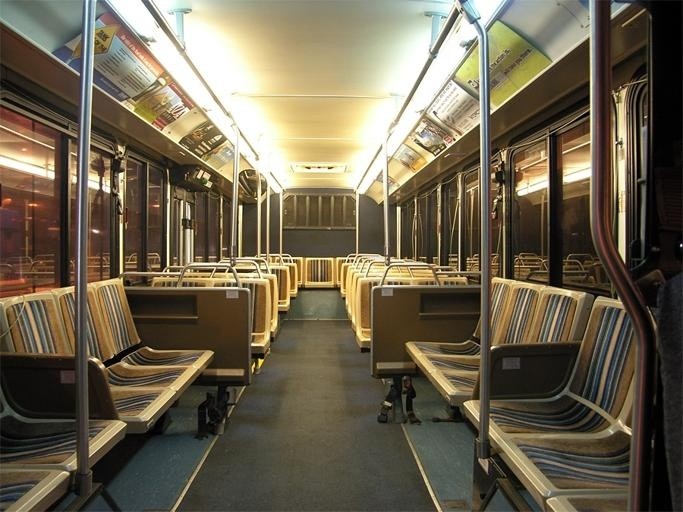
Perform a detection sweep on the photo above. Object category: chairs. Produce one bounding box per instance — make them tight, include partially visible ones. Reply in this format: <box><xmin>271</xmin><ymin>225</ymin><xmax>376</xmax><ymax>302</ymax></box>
<box><xmin>445</xmin><ymin>249</ymin><xmax>609</xmax><ymax>283</ymax></box>
<box><xmin>459</xmin><ymin>295</ymin><xmax>657</xmax><ymax>512</ymax></box>
<box><xmin>143</xmin><ymin>252</ymin><xmax>302</xmax><ymax>430</ymax></box>
<box><xmin>1</xmin><ymin>384</ymin><xmax>128</xmax><ymax>511</ymax></box>
<box><xmin>303</xmin><ymin>257</ymin><xmax>336</xmax><ymax>288</ymax></box>
<box><xmin>0</xmin><ymin>276</ymin><xmax>215</xmax><ymax>434</ymax></box>
<box><xmin>401</xmin><ymin>271</ymin><xmax>600</xmax><ymax>437</ymax></box>
<box><xmin>0</xmin><ymin>249</ymin><xmax>164</xmax><ymax>289</ymax></box>
<box><xmin>344</xmin><ymin>254</ymin><xmax>473</xmax><ymax>433</ymax></box>
